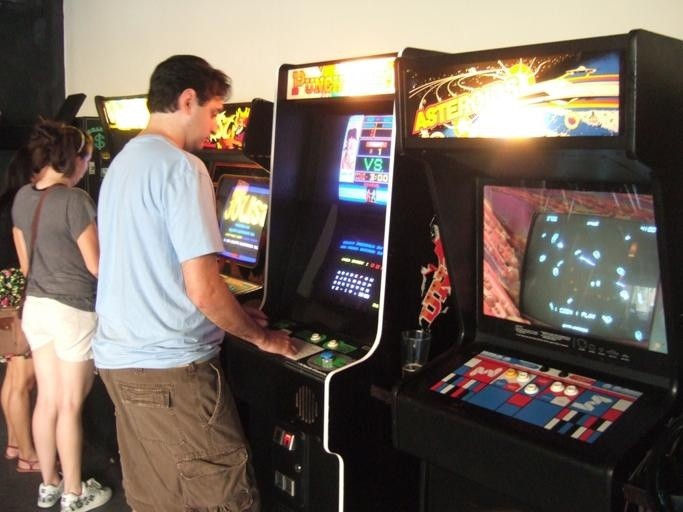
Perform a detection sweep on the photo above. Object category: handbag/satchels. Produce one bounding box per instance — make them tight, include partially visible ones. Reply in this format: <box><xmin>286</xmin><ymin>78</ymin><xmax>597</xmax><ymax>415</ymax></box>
<box><xmin>0</xmin><ymin>307</ymin><xmax>30</xmax><ymax>356</ymax></box>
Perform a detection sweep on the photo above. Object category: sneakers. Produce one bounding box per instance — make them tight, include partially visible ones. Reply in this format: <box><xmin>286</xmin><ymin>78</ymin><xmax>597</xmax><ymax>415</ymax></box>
<box><xmin>61</xmin><ymin>478</ymin><xmax>112</xmax><ymax>512</ymax></box>
<box><xmin>38</xmin><ymin>472</ymin><xmax>63</xmax><ymax>508</ymax></box>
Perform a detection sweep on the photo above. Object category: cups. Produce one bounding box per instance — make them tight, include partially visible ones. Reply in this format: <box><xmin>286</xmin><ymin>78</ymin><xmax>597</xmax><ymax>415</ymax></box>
<box><xmin>400</xmin><ymin>331</ymin><xmax>432</xmax><ymax>374</ymax></box>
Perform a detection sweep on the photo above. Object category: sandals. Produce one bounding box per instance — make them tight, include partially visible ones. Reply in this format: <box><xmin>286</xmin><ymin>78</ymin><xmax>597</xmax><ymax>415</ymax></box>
<box><xmin>16</xmin><ymin>457</ymin><xmax>59</xmax><ymax>473</ymax></box>
<box><xmin>4</xmin><ymin>445</ymin><xmax>18</xmax><ymax>459</ymax></box>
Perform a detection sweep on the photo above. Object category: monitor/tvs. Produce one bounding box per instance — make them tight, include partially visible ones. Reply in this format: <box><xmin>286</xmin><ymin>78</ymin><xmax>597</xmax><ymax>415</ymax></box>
<box><xmin>472</xmin><ymin>174</ymin><xmax>667</xmax><ymax>384</ymax></box>
<box><xmin>302</xmin><ymin>212</ymin><xmax>386</xmax><ymax>326</ymax></box>
<box><xmin>213</xmin><ymin>180</ymin><xmax>265</xmax><ymax>270</ymax></box>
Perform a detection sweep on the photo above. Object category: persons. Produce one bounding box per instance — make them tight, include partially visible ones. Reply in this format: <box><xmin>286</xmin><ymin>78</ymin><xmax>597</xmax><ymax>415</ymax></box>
<box><xmin>88</xmin><ymin>54</ymin><xmax>297</xmax><ymax>510</ymax></box>
<box><xmin>0</xmin><ymin>145</ymin><xmax>49</xmax><ymax>476</ymax></box>
<box><xmin>11</xmin><ymin>120</ymin><xmax>112</xmax><ymax>512</ymax></box>
